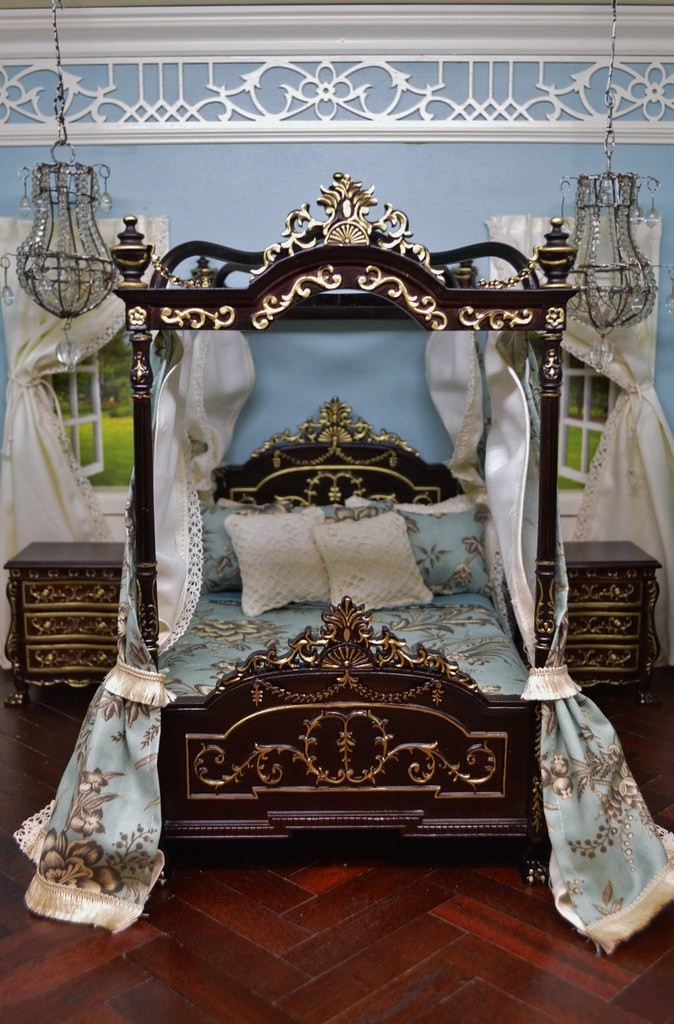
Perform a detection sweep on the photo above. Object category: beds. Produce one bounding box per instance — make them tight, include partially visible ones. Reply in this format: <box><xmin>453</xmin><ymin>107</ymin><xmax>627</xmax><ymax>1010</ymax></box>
<box><xmin>107</xmin><ymin>168</ymin><xmax>589</xmax><ymax>889</ymax></box>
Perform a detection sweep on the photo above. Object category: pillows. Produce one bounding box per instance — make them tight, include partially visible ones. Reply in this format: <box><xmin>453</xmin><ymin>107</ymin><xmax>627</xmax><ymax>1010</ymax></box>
<box><xmin>312</xmin><ymin>510</ymin><xmax>434</xmax><ymax>616</ymax></box>
<box><xmin>222</xmin><ymin>506</ymin><xmax>333</xmax><ymax>618</ymax></box>
<box><xmin>370</xmin><ymin>503</ymin><xmax>494</xmax><ymax>601</ymax></box>
<box><xmin>196</xmin><ymin>500</ymin><xmax>342</xmax><ymax>597</ymax></box>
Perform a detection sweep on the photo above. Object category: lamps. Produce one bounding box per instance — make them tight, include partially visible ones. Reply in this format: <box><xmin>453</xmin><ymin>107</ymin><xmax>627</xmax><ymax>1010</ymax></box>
<box><xmin>560</xmin><ymin>0</ymin><xmax>674</xmax><ymax>375</ymax></box>
<box><xmin>0</xmin><ymin>0</ymin><xmax>120</xmax><ymax>374</ymax></box>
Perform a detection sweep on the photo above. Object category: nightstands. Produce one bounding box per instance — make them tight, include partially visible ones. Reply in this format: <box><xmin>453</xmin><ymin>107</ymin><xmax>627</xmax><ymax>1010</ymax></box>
<box><xmin>561</xmin><ymin>540</ymin><xmax>664</xmax><ymax>701</ymax></box>
<box><xmin>3</xmin><ymin>537</ymin><xmax>128</xmax><ymax>704</ymax></box>
